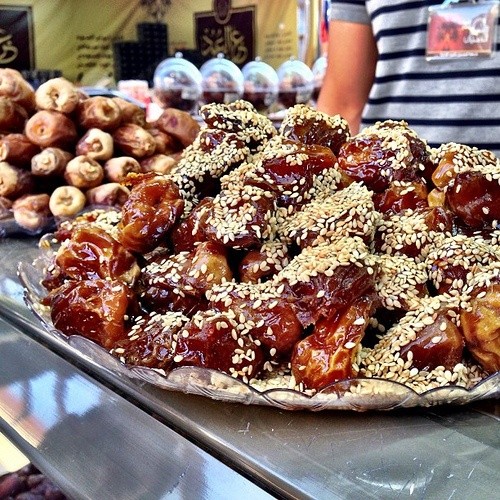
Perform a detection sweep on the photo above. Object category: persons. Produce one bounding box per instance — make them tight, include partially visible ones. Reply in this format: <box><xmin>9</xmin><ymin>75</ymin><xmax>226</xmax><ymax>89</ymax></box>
<box><xmin>34</xmin><ymin>401</ymin><xmax>179</xmax><ymax>500</ymax></box>
<box><xmin>312</xmin><ymin>0</ymin><xmax>500</xmax><ymax>159</ymax></box>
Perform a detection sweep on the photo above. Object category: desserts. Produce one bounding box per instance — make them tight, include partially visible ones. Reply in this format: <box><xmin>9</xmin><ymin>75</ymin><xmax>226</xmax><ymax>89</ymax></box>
<box><xmin>40</xmin><ymin>98</ymin><xmax>500</xmax><ymax>393</ymax></box>
